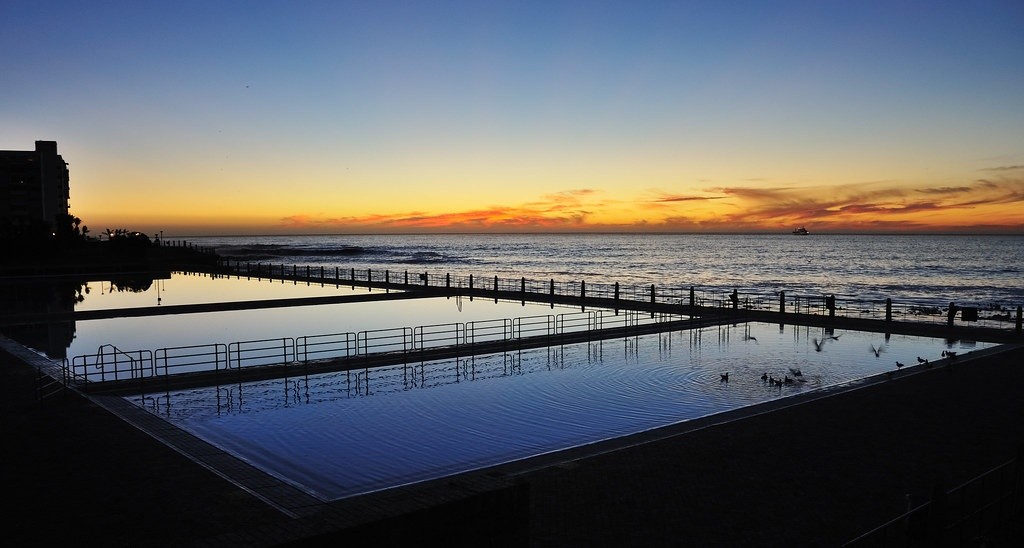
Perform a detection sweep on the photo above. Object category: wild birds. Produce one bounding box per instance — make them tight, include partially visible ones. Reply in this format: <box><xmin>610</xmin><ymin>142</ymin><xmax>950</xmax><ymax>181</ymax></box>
<box><xmin>812</xmin><ymin>334</ymin><xmax>957</xmax><ymax>368</ymax></box>
<box><xmin>720</xmin><ymin>368</ymin><xmax>808</xmax><ymax>389</ymax></box>
<box><xmin>749</xmin><ymin>337</ymin><xmax>756</xmax><ymax>342</ymax></box>
